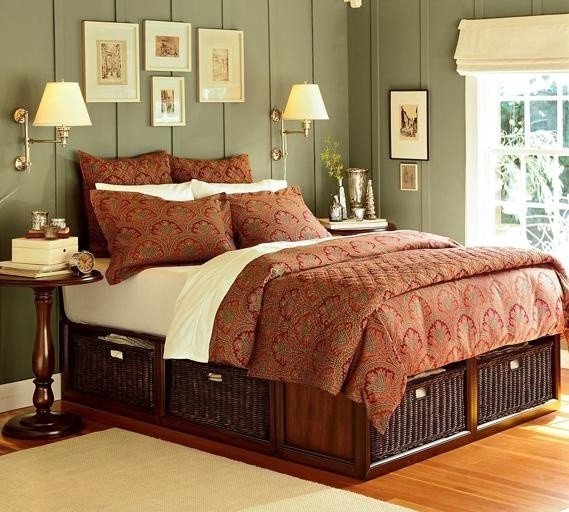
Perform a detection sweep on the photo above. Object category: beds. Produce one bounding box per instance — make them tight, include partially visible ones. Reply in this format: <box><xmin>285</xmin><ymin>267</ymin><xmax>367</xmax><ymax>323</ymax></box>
<box><xmin>61</xmin><ymin>251</ymin><xmax>562</xmax><ymax>481</ymax></box>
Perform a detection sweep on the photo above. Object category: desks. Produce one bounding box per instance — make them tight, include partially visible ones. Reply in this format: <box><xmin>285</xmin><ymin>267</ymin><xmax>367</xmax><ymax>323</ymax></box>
<box><xmin>0</xmin><ymin>270</ymin><xmax>103</xmax><ymax>439</ymax></box>
<box><xmin>326</xmin><ymin>223</ymin><xmax>397</xmax><ymax>235</ymax></box>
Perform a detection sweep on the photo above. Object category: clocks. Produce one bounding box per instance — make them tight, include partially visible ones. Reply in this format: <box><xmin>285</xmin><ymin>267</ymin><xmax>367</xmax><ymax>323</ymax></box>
<box><xmin>70</xmin><ymin>251</ymin><xmax>95</xmax><ymax>276</ymax></box>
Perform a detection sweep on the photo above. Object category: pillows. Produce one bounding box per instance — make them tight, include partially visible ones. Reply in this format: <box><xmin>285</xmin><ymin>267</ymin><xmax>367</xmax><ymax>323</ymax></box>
<box><xmin>191</xmin><ymin>177</ymin><xmax>288</xmax><ymax>199</ymax></box>
<box><xmin>94</xmin><ymin>180</ymin><xmax>194</xmax><ymax>201</ymax></box>
<box><xmin>89</xmin><ymin>190</ymin><xmax>236</xmax><ymax>286</ymax></box>
<box><xmin>228</xmin><ymin>185</ymin><xmax>332</xmax><ymax>248</ymax></box>
<box><xmin>78</xmin><ymin>149</ymin><xmax>174</xmax><ymax>258</ymax></box>
<box><xmin>168</xmin><ymin>153</ymin><xmax>254</xmax><ymax>183</ymax></box>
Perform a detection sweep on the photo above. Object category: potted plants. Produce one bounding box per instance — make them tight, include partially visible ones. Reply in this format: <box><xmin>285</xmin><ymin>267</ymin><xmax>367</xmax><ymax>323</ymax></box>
<box><xmin>320</xmin><ymin>136</ymin><xmax>348</xmax><ymax>220</ymax></box>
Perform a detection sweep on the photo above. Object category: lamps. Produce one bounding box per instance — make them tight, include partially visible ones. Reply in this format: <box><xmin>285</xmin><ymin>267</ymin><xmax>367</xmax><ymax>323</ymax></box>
<box><xmin>13</xmin><ymin>79</ymin><xmax>93</xmax><ymax>172</ymax></box>
<box><xmin>272</xmin><ymin>82</ymin><xmax>330</xmax><ymax>161</ymax></box>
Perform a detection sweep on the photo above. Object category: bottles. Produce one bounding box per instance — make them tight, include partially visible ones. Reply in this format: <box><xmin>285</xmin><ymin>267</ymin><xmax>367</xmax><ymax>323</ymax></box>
<box><xmin>329</xmin><ymin>195</ymin><xmax>342</xmax><ymax>222</ymax></box>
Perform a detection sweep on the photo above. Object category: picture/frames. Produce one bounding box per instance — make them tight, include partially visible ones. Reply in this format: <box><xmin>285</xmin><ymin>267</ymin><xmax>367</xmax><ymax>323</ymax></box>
<box><xmin>82</xmin><ymin>19</ymin><xmax>141</xmax><ymax>103</ymax></box>
<box><xmin>150</xmin><ymin>75</ymin><xmax>186</xmax><ymax>128</ymax></box>
<box><xmin>196</xmin><ymin>27</ymin><xmax>245</xmax><ymax>104</ymax></box>
<box><xmin>142</xmin><ymin>18</ymin><xmax>192</xmax><ymax>73</ymax></box>
<box><xmin>399</xmin><ymin>163</ymin><xmax>418</xmax><ymax>191</ymax></box>
<box><xmin>388</xmin><ymin>88</ymin><xmax>430</xmax><ymax>161</ymax></box>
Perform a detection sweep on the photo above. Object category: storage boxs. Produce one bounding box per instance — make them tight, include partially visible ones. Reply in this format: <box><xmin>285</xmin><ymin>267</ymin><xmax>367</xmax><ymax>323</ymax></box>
<box><xmin>165</xmin><ymin>353</ymin><xmax>271</xmax><ymax>443</ymax></box>
<box><xmin>69</xmin><ymin>335</ymin><xmax>158</xmax><ymax>409</ymax></box>
<box><xmin>477</xmin><ymin>335</ymin><xmax>557</xmax><ymax>428</ymax></box>
<box><xmin>368</xmin><ymin>359</ymin><xmax>467</xmax><ymax>467</ymax></box>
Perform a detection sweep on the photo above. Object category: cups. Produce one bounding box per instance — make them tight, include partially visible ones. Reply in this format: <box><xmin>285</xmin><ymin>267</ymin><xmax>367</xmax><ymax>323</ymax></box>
<box><xmin>352</xmin><ymin>207</ymin><xmax>366</xmax><ymax>221</ymax></box>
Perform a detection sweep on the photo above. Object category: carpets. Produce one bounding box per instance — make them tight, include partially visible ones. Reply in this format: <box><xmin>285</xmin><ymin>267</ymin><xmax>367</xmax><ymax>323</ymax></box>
<box><xmin>0</xmin><ymin>428</ymin><xmax>418</xmax><ymax>512</ymax></box>
